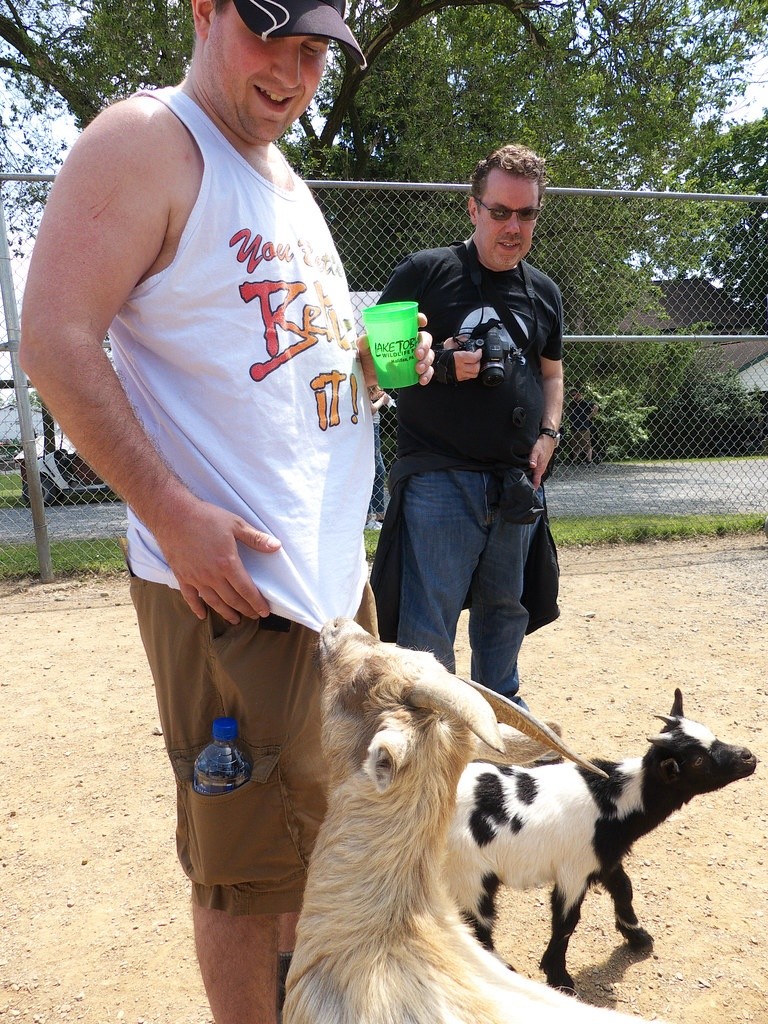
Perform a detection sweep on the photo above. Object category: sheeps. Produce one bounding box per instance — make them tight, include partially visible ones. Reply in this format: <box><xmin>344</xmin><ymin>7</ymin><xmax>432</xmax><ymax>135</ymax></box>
<box><xmin>283</xmin><ymin>619</ymin><xmax>768</xmax><ymax>1024</ymax></box>
<box><xmin>444</xmin><ymin>686</ymin><xmax>758</xmax><ymax>998</ymax></box>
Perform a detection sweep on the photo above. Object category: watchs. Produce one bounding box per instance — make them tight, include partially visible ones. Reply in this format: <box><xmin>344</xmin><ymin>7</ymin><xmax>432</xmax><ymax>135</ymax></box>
<box><xmin>539</xmin><ymin>427</ymin><xmax>561</xmax><ymax>449</ymax></box>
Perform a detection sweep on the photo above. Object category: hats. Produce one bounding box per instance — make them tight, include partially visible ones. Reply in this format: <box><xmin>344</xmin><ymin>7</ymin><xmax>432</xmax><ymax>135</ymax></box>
<box><xmin>233</xmin><ymin>0</ymin><xmax>367</xmax><ymax>69</ymax></box>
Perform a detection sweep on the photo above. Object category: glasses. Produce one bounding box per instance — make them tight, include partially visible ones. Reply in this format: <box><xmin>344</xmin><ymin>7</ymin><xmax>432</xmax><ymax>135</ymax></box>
<box><xmin>473</xmin><ymin>196</ymin><xmax>540</xmax><ymax>221</ymax></box>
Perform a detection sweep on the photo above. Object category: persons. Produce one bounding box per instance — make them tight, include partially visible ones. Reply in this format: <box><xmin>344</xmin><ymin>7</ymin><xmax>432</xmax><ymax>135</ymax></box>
<box><xmin>372</xmin><ymin>142</ymin><xmax>564</xmax><ymax>713</ymax></box>
<box><xmin>22</xmin><ymin>4</ymin><xmax>436</xmax><ymax>1024</ymax></box>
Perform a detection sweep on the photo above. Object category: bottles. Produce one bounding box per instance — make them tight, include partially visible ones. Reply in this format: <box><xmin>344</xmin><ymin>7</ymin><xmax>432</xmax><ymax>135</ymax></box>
<box><xmin>193</xmin><ymin>718</ymin><xmax>253</xmax><ymax>796</ymax></box>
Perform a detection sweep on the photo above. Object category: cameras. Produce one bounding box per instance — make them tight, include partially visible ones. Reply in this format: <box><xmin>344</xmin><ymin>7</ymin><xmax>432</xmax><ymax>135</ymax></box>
<box><xmin>460</xmin><ymin>331</ymin><xmax>510</xmax><ymax>386</ymax></box>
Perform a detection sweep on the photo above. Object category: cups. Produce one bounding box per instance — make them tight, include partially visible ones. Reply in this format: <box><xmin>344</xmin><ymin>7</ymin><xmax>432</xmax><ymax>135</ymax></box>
<box><xmin>361</xmin><ymin>301</ymin><xmax>420</xmax><ymax>389</ymax></box>
